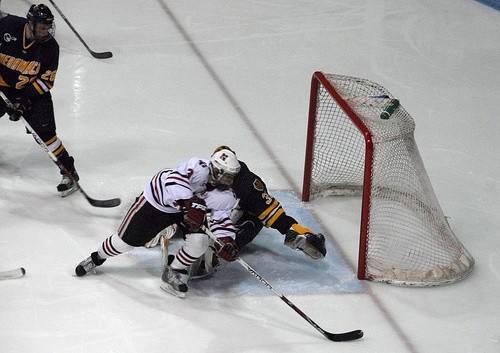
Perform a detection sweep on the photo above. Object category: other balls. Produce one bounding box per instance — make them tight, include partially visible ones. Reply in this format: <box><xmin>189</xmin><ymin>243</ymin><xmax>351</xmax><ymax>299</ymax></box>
<box><xmin>0</xmin><ymin>89</ymin><xmax>121</xmax><ymax>208</ymax></box>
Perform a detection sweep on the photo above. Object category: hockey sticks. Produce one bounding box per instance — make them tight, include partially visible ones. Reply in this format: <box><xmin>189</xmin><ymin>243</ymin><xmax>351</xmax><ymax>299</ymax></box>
<box><xmin>199</xmin><ymin>224</ymin><xmax>366</xmax><ymax>343</ymax></box>
<box><xmin>50</xmin><ymin>0</ymin><xmax>114</xmax><ymax>60</ymax></box>
<box><xmin>0</xmin><ymin>267</ymin><xmax>26</xmax><ymax>281</ymax></box>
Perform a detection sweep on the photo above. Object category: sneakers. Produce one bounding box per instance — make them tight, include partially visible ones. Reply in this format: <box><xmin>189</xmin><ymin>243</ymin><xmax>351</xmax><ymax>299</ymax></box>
<box><xmin>160</xmin><ymin>255</ymin><xmax>188</xmax><ymax>297</ymax></box>
<box><xmin>75</xmin><ymin>251</ymin><xmax>107</xmax><ymax>277</ymax></box>
<box><xmin>57</xmin><ymin>156</ymin><xmax>80</xmax><ymax>196</ymax></box>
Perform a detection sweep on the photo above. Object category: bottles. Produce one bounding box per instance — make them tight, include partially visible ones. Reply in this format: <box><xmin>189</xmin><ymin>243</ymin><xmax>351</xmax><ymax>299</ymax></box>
<box><xmin>380</xmin><ymin>98</ymin><xmax>399</xmax><ymax>119</ymax></box>
<box><xmin>0</xmin><ymin>267</ymin><xmax>25</xmax><ymax>280</ymax></box>
<box><xmin>369</xmin><ymin>94</ymin><xmax>390</xmax><ymax>99</ymax></box>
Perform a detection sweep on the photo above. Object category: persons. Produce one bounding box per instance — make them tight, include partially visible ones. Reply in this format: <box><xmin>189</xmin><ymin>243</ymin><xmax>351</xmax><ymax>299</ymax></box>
<box><xmin>0</xmin><ymin>3</ymin><xmax>80</xmax><ymax>199</ymax></box>
<box><xmin>74</xmin><ymin>145</ymin><xmax>328</xmax><ymax>299</ymax></box>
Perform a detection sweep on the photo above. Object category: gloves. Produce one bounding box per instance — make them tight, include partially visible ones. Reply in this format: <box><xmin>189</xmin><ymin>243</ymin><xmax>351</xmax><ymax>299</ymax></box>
<box><xmin>184</xmin><ymin>196</ymin><xmax>207</xmax><ymax>231</ymax></box>
<box><xmin>215</xmin><ymin>237</ymin><xmax>239</xmax><ymax>262</ymax></box>
<box><xmin>6</xmin><ymin>97</ymin><xmax>32</xmax><ymax>120</ymax></box>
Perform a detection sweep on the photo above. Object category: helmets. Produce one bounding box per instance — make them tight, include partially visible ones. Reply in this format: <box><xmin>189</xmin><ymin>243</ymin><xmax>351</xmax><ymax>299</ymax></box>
<box><xmin>26</xmin><ymin>4</ymin><xmax>54</xmax><ymax>25</ymax></box>
<box><xmin>211</xmin><ymin>146</ymin><xmax>236</xmax><ymax>158</ymax></box>
<box><xmin>210</xmin><ymin>150</ymin><xmax>241</xmax><ymax>175</ymax></box>
